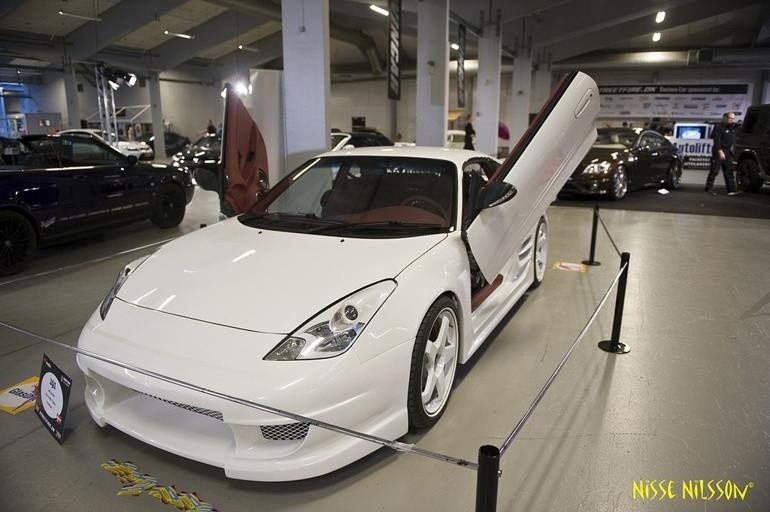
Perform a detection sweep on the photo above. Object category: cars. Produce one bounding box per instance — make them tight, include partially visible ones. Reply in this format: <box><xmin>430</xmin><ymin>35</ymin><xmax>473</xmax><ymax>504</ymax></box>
<box><xmin>555</xmin><ymin>123</ymin><xmax>686</xmax><ymax>201</ymax></box>
<box><xmin>326</xmin><ymin>122</ymin><xmax>479</xmax><ymax>151</ymax></box>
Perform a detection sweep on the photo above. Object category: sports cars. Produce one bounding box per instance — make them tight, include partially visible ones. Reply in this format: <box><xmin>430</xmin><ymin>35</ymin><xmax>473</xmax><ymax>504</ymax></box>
<box><xmin>0</xmin><ymin>122</ymin><xmax>222</xmax><ymax>282</ymax></box>
<box><xmin>71</xmin><ymin>63</ymin><xmax>602</xmax><ymax>486</ymax></box>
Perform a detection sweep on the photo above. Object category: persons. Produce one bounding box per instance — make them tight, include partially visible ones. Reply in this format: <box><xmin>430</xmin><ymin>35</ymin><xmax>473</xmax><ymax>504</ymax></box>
<box><xmin>216</xmin><ymin>123</ymin><xmax>222</xmax><ymax>136</ymax></box>
<box><xmin>621</xmin><ymin>121</ymin><xmax>670</xmax><ymax>136</ymax></box>
<box><xmin>464</xmin><ymin>113</ymin><xmax>476</xmax><ymax>150</ymax></box>
<box><xmin>207</xmin><ymin>118</ymin><xmax>216</xmax><ymax>134</ymax></box>
<box><xmin>702</xmin><ymin>112</ymin><xmax>744</xmax><ymax>197</ymax></box>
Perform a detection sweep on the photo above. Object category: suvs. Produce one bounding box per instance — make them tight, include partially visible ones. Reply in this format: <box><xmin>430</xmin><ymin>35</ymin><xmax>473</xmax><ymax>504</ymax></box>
<box><xmin>732</xmin><ymin>102</ymin><xmax>770</xmax><ymax>194</ymax></box>
